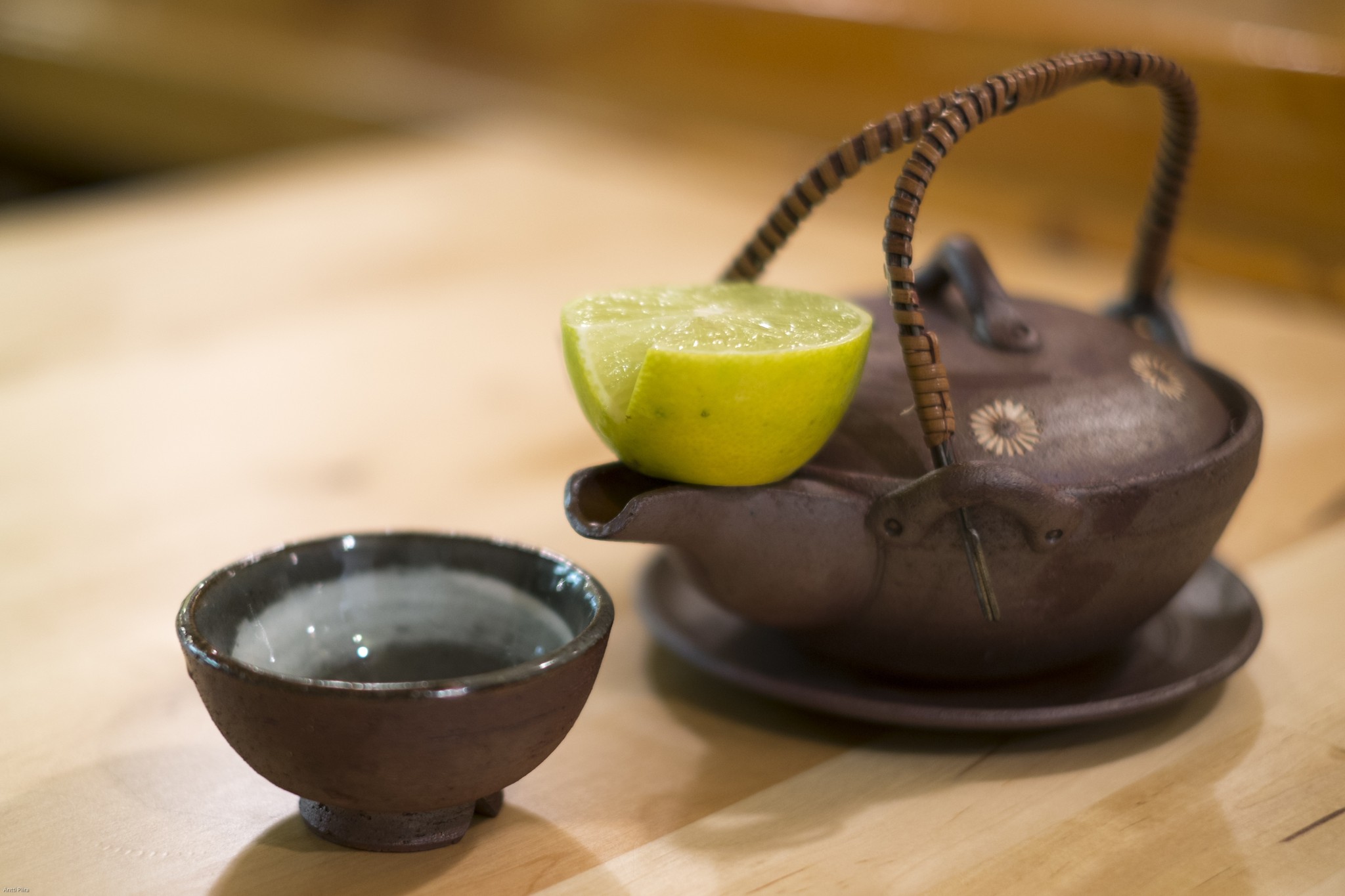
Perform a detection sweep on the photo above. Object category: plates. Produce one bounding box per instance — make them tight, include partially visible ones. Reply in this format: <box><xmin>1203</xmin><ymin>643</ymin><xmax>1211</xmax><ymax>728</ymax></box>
<box><xmin>636</xmin><ymin>541</ymin><xmax>1264</xmax><ymax>729</ymax></box>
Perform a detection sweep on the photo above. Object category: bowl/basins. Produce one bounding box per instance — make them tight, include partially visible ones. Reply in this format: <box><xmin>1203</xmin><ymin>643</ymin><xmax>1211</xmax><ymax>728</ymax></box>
<box><xmin>176</xmin><ymin>532</ymin><xmax>611</xmax><ymax>853</ymax></box>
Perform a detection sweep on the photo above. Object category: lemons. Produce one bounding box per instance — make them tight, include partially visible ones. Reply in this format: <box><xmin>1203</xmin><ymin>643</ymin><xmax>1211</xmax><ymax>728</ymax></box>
<box><xmin>558</xmin><ymin>285</ymin><xmax>876</xmax><ymax>488</ymax></box>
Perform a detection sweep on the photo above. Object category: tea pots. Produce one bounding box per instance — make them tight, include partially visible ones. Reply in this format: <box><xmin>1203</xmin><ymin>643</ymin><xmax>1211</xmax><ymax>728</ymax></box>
<box><xmin>562</xmin><ymin>48</ymin><xmax>1266</xmax><ymax>685</ymax></box>
